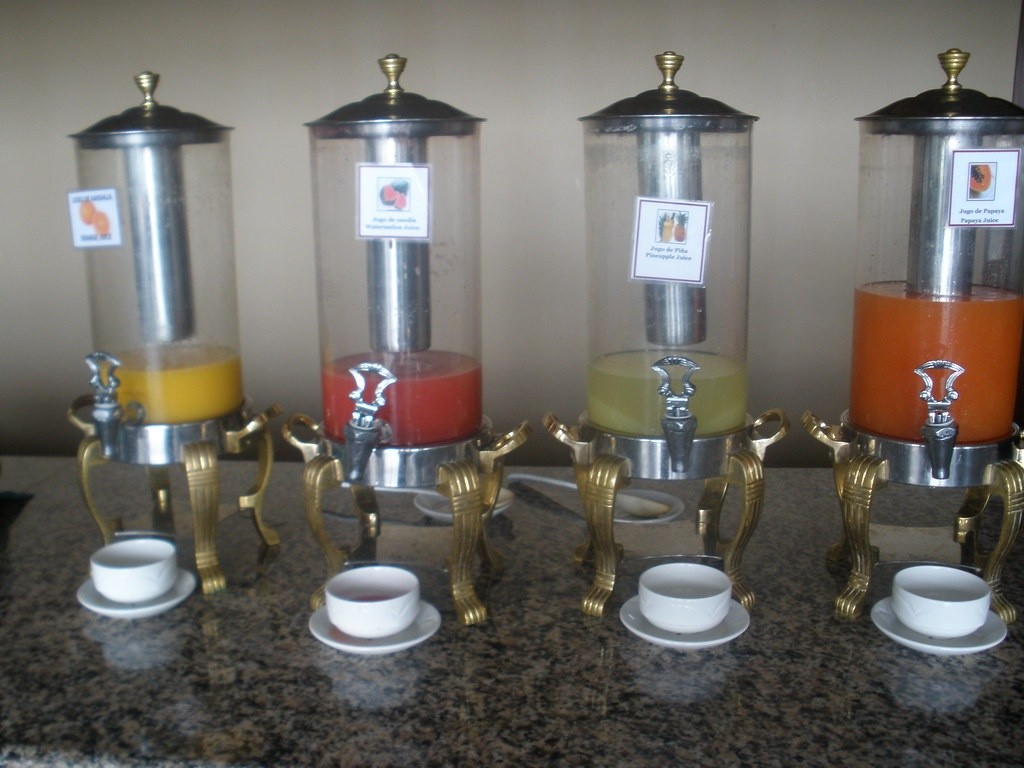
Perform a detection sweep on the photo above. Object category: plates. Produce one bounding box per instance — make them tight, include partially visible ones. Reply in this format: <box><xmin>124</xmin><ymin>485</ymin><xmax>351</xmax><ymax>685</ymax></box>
<box><xmin>619</xmin><ymin>594</ymin><xmax>750</xmax><ymax>647</ymax></box>
<box><xmin>870</xmin><ymin>596</ymin><xmax>1009</xmax><ymax>656</ymax></box>
<box><xmin>612</xmin><ymin>488</ymin><xmax>686</xmax><ymax>524</ymax></box>
<box><xmin>413</xmin><ymin>486</ymin><xmax>515</xmax><ymax>523</ymax></box>
<box><xmin>76</xmin><ymin>565</ymin><xmax>197</xmax><ymax>619</ymax></box>
<box><xmin>309</xmin><ymin>600</ymin><xmax>441</xmax><ymax>653</ymax></box>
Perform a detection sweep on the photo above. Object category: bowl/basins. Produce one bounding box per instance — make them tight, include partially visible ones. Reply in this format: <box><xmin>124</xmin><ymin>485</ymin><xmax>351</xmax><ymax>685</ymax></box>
<box><xmin>90</xmin><ymin>538</ymin><xmax>177</xmax><ymax>605</ymax></box>
<box><xmin>324</xmin><ymin>565</ymin><xmax>421</xmax><ymax>639</ymax></box>
<box><xmin>891</xmin><ymin>565</ymin><xmax>991</xmax><ymax>638</ymax></box>
<box><xmin>637</xmin><ymin>562</ymin><xmax>732</xmax><ymax>634</ymax></box>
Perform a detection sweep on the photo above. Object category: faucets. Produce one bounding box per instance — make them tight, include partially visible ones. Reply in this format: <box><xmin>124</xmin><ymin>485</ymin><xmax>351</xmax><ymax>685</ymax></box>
<box><xmin>651</xmin><ymin>355</ymin><xmax>700</xmax><ymax>474</ymax></box>
<box><xmin>82</xmin><ymin>351</ymin><xmax>124</xmax><ymax>461</ymax></box>
<box><xmin>344</xmin><ymin>363</ymin><xmax>398</xmax><ymax>482</ymax></box>
<box><xmin>913</xmin><ymin>360</ymin><xmax>966</xmax><ymax>479</ymax></box>
